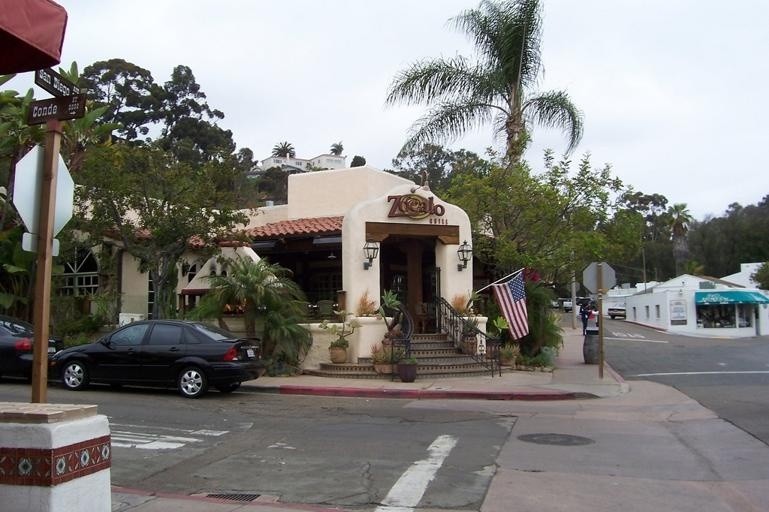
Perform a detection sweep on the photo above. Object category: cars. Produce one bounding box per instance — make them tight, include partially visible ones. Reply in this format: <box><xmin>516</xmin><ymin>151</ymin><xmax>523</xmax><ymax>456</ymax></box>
<box><xmin>0</xmin><ymin>315</ymin><xmax>262</xmax><ymax>399</ymax></box>
<box><xmin>554</xmin><ymin>297</ymin><xmax>626</xmax><ymax>320</ymax></box>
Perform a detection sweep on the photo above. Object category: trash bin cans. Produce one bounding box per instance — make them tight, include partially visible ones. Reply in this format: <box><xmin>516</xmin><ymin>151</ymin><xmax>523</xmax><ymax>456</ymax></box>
<box><xmin>583</xmin><ymin>330</ymin><xmax>600</xmax><ymax>364</ymax></box>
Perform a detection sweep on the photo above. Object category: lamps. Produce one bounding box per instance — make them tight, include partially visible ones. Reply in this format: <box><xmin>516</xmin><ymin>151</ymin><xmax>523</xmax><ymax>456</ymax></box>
<box><xmin>457</xmin><ymin>240</ymin><xmax>473</xmax><ymax>271</ymax></box>
<box><xmin>363</xmin><ymin>239</ymin><xmax>379</xmax><ymax>270</ymax></box>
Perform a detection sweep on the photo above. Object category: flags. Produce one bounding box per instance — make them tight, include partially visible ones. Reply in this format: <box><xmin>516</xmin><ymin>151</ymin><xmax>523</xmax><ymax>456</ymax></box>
<box><xmin>492</xmin><ymin>272</ymin><xmax>530</xmax><ymax>342</ymax></box>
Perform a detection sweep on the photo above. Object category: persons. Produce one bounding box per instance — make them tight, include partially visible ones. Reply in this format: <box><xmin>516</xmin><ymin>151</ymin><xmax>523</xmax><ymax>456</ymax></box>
<box><xmin>587</xmin><ymin>306</ymin><xmax>599</xmax><ymax>323</ymax></box>
<box><xmin>580</xmin><ymin>303</ymin><xmax>590</xmax><ymax>335</ymax></box>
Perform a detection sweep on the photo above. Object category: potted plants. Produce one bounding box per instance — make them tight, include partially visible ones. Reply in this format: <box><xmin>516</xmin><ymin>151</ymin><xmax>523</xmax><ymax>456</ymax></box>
<box><xmin>318</xmin><ymin>289</ymin><xmax>520</xmax><ymax>382</ymax></box>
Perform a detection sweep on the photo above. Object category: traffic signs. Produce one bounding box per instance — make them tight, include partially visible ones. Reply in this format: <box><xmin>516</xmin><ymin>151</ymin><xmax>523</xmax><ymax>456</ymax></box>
<box><xmin>35</xmin><ymin>64</ymin><xmax>82</xmax><ymax>97</ymax></box>
<box><xmin>26</xmin><ymin>93</ymin><xmax>86</xmax><ymax>126</ymax></box>
